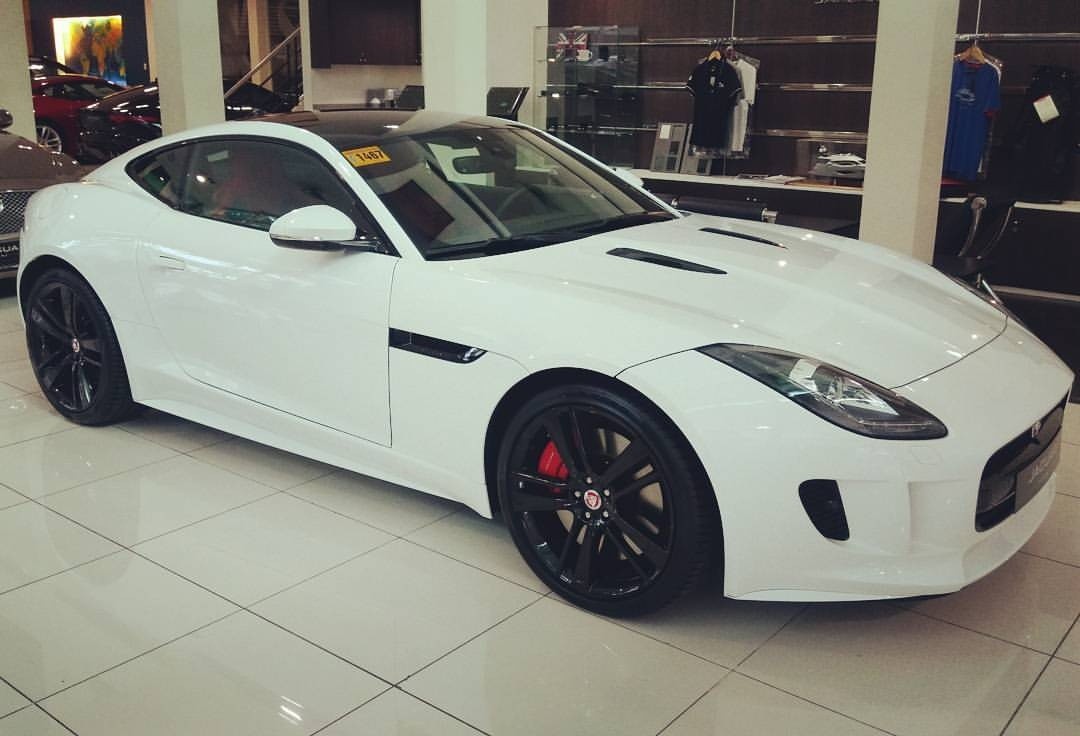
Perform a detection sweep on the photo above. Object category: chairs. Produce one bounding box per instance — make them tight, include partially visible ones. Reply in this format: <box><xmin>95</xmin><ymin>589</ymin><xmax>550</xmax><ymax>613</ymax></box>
<box><xmin>933</xmin><ymin>181</ymin><xmax>1019</xmax><ymax>304</ymax></box>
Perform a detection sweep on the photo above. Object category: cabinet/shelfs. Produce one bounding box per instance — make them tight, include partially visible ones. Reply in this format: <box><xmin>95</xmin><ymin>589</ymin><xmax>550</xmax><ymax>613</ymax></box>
<box><xmin>590</xmin><ymin>31</ymin><xmax>1080</xmax><ymax>147</ymax></box>
<box><xmin>532</xmin><ymin>21</ymin><xmax>640</xmax><ymax>173</ymax></box>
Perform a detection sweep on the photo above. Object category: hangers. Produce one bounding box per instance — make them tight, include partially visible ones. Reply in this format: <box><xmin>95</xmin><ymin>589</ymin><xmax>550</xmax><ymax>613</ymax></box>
<box><xmin>707</xmin><ymin>38</ymin><xmax>722</xmax><ymax>62</ymax></box>
<box><xmin>958</xmin><ymin>39</ymin><xmax>986</xmax><ymax>64</ymax></box>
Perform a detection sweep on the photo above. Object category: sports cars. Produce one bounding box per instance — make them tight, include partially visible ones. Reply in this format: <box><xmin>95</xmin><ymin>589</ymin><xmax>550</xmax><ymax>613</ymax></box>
<box><xmin>0</xmin><ymin>53</ymin><xmax>303</xmax><ymax>296</ymax></box>
<box><xmin>17</xmin><ymin>109</ymin><xmax>1074</xmax><ymax>616</ymax></box>
